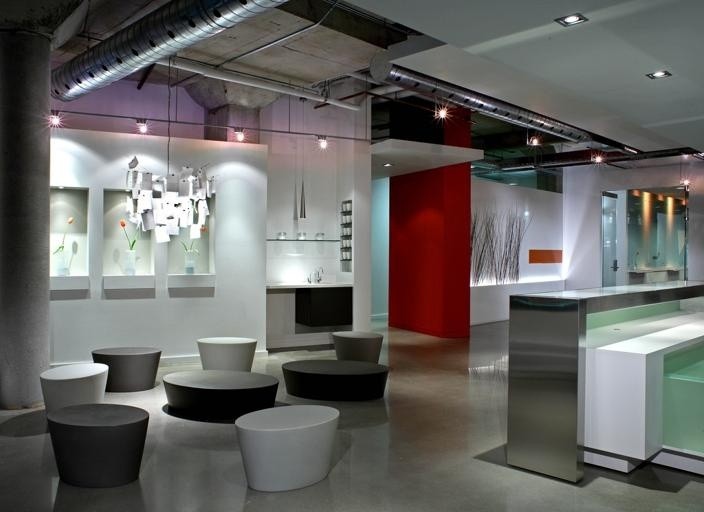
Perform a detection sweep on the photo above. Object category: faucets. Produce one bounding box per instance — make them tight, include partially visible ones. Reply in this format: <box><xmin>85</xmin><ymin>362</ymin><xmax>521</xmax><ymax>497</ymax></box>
<box><xmin>316</xmin><ymin>265</ymin><xmax>323</xmax><ymax>284</ymax></box>
<box><xmin>307</xmin><ymin>271</ymin><xmax>315</xmax><ymax>283</ymax></box>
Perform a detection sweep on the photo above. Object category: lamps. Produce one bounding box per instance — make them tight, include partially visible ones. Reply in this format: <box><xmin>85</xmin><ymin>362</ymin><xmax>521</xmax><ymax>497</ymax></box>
<box><xmin>124</xmin><ymin>52</ymin><xmax>218</xmax><ymax>246</ymax></box>
<box><xmin>52</xmin><ymin>112</ymin><xmax>59</xmax><ymax>118</ymax></box>
<box><xmin>299</xmin><ymin>97</ymin><xmax>307</xmax><ymax>218</ymax></box>
<box><xmin>233</xmin><ymin>127</ymin><xmax>244</xmax><ymax>133</ymax></box>
<box><xmin>317</xmin><ymin>135</ymin><xmax>327</xmax><ymax>142</ymax></box>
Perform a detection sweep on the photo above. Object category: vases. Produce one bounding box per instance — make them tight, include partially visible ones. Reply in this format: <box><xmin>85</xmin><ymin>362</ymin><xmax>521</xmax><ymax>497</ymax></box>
<box><xmin>184</xmin><ymin>249</ymin><xmax>195</xmax><ymax>274</ymax></box>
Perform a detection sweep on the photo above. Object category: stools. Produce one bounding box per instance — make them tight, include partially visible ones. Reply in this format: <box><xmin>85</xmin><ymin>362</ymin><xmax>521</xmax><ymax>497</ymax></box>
<box><xmin>48</xmin><ymin>402</ymin><xmax>150</xmax><ymax>487</ymax></box>
<box><xmin>198</xmin><ymin>336</ymin><xmax>258</xmax><ymax>371</ymax></box>
<box><xmin>91</xmin><ymin>347</ymin><xmax>162</xmax><ymax>392</ymax></box>
<box><xmin>333</xmin><ymin>332</ymin><xmax>384</xmax><ymax>363</ymax></box>
<box><xmin>39</xmin><ymin>364</ymin><xmax>108</xmax><ymax>408</ymax></box>
<box><xmin>236</xmin><ymin>406</ymin><xmax>340</xmax><ymax>492</ymax></box>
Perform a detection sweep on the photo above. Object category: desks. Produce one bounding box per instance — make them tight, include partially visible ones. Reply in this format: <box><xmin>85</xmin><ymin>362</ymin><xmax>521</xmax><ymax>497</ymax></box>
<box><xmin>282</xmin><ymin>359</ymin><xmax>391</xmax><ymax>400</ymax></box>
<box><xmin>163</xmin><ymin>369</ymin><xmax>279</xmax><ymax>423</ymax></box>
<box><xmin>501</xmin><ymin>280</ymin><xmax>704</xmax><ymax>486</ymax></box>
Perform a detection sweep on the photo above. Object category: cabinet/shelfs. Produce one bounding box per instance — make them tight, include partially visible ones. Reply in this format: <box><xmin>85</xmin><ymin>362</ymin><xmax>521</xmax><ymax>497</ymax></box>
<box><xmin>339</xmin><ymin>210</ymin><xmax>352</xmax><ymax>261</ymax></box>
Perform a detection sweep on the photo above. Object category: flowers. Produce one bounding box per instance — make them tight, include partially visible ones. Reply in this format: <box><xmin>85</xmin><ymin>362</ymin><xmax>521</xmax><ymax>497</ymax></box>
<box><xmin>177</xmin><ymin>225</ymin><xmax>208</xmax><ymax>251</ymax></box>
<box><xmin>117</xmin><ymin>219</ymin><xmax>138</xmax><ymax>250</ymax></box>
<box><xmin>51</xmin><ymin>217</ymin><xmax>76</xmax><ymax>256</ymax></box>
